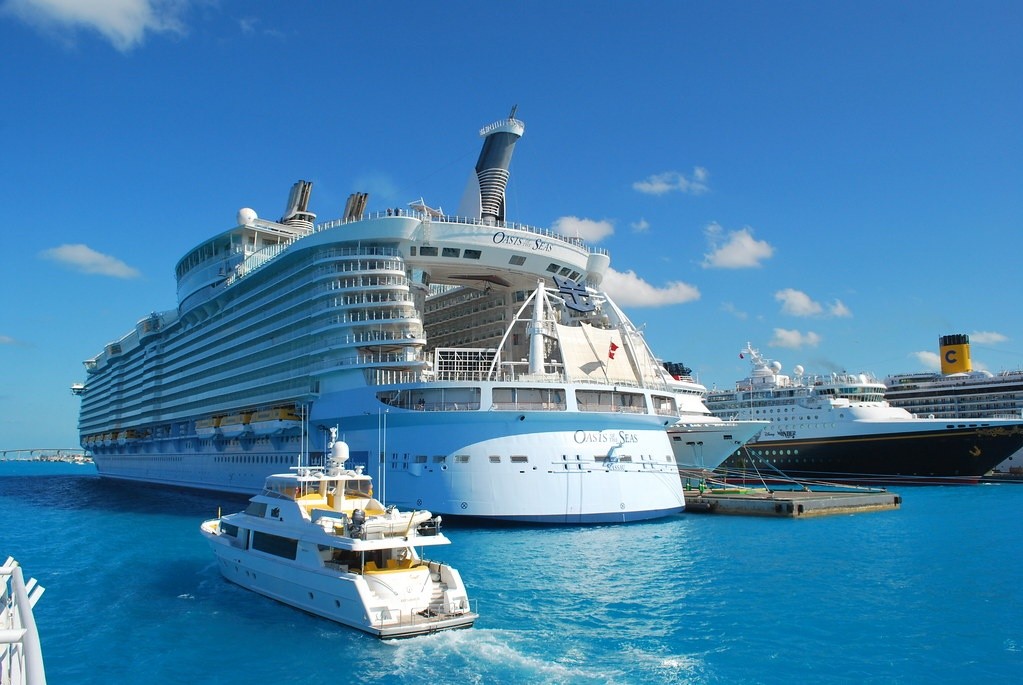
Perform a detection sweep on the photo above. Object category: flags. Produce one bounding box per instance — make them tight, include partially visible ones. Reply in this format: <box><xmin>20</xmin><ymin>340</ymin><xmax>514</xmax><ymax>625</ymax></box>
<box><xmin>609</xmin><ymin>352</ymin><xmax>615</xmax><ymax>359</ymax></box>
<box><xmin>610</xmin><ymin>343</ymin><xmax>618</xmax><ymax>351</ymax></box>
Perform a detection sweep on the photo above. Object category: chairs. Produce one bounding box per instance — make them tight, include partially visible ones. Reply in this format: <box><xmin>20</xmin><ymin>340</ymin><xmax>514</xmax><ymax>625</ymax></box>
<box><xmin>367</xmin><ymin>558</ymin><xmax>413</xmax><ymax>571</ymax></box>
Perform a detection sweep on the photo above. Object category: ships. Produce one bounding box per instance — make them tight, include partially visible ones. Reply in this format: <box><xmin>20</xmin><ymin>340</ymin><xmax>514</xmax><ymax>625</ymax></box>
<box><xmin>654</xmin><ymin>333</ymin><xmax>1023</xmax><ymax>490</ymax></box>
<box><xmin>68</xmin><ymin>103</ymin><xmax>685</xmax><ymax>529</ymax></box>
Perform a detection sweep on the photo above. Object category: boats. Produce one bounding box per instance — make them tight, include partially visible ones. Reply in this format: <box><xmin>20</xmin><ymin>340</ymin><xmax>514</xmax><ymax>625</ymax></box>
<box><xmin>88</xmin><ymin>434</ymin><xmax>97</xmax><ymax>450</ymax></box>
<box><xmin>250</xmin><ymin>406</ymin><xmax>307</xmax><ymax>438</ymax></box>
<box><xmin>194</xmin><ymin>417</ymin><xmax>227</xmax><ymax>439</ymax></box>
<box><xmin>219</xmin><ymin>412</ymin><xmax>255</xmax><ymax>439</ymax></box>
<box><xmin>116</xmin><ymin>429</ymin><xmax>149</xmax><ymax>448</ymax></box>
<box><xmin>103</xmin><ymin>430</ymin><xmax>123</xmax><ymax>449</ymax></box>
<box><xmin>94</xmin><ymin>433</ymin><xmax>105</xmax><ymax>450</ymax></box>
<box><xmin>200</xmin><ymin>401</ymin><xmax>481</xmax><ymax>641</ymax></box>
<box><xmin>81</xmin><ymin>435</ymin><xmax>91</xmax><ymax>450</ymax></box>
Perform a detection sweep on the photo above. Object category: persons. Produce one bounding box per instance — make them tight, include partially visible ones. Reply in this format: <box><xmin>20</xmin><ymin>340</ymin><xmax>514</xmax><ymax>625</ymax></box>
<box><xmin>394</xmin><ymin>208</ymin><xmax>402</xmax><ymax>216</ymax></box>
<box><xmin>387</xmin><ymin>208</ymin><xmax>392</xmax><ymax>216</ymax></box>
<box><xmin>418</xmin><ymin>398</ymin><xmax>425</xmax><ymax>406</ymax></box>
